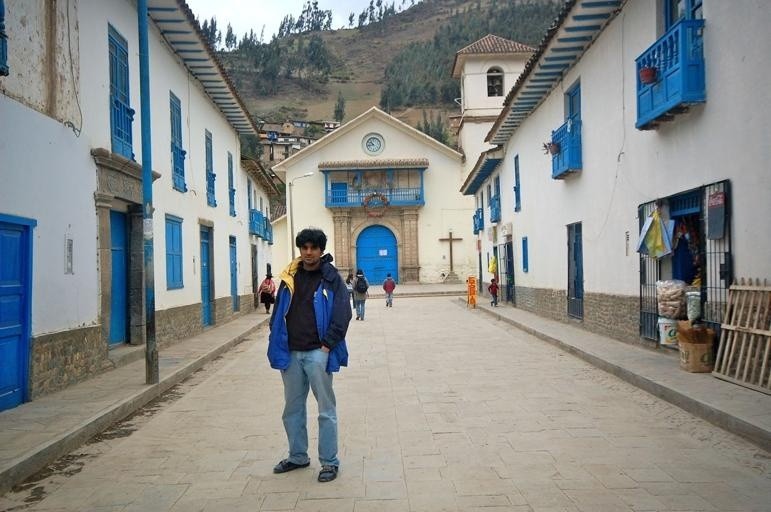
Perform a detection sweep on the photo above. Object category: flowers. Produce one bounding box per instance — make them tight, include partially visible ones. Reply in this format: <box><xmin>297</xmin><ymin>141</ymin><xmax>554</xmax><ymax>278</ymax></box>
<box><xmin>361</xmin><ymin>191</ymin><xmax>389</xmax><ymax>218</ymax></box>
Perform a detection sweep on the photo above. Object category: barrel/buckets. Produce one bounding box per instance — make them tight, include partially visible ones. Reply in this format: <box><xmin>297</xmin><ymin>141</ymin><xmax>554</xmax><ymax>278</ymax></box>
<box><xmin>656</xmin><ymin>317</ymin><xmax>680</xmax><ymax>345</ymax></box>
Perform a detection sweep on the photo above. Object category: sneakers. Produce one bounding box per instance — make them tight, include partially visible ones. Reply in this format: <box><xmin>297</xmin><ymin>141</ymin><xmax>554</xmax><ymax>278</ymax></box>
<box><xmin>356</xmin><ymin>316</ymin><xmax>364</xmax><ymax>320</ymax></box>
<box><xmin>274</xmin><ymin>458</ymin><xmax>310</xmax><ymax>473</ymax></box>
<box><xmin>386</xmin><ymin>302</ymin><xmax>392</xmax><ymax>307</ymax></box>
<box><xmin>491</xmin><ymin>302</ymin><xmax>498</xmax><ymax>307</ymax></box>
<box><xmin>318</xmin><ymin>464</ymin><xmax>338</xmax><ymax>481</ymax></box>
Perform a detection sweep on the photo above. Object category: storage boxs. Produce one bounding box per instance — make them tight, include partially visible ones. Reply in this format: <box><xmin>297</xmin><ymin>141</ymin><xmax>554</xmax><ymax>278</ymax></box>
<box><xmin>677</xmin><ymin>340</ymin><xmax>715</xmax><ymax>373</ymax></box>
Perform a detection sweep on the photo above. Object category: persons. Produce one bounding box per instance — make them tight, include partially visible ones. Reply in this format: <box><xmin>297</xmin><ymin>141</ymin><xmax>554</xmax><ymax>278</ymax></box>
<box><xmin>344</xmin><ymin>273</ymin><xmax>356</xmax><ymax>310</ymax></box>
<box><xmin>352</xmin><ymin>268</ymin><xmax>369</xmax><ymax>321</ymax></box>
<box><xmin>382</xmin><ymin>273</ymin><xmax>396</xmax><ymax>307</ymax></box>
<box><xmin>257</xmin><ymin>272</ymin><xmax>276</xmax><ymax>315</ymax></box>
<box><xmin>267</xmin><ymin>228</ymin><xmax>353</xmax><ymax>483</ymax></box>
<box><xmin>487</xmin><ymin>278</ymin><xmax>500</xmax><ymax>307</ymax></box>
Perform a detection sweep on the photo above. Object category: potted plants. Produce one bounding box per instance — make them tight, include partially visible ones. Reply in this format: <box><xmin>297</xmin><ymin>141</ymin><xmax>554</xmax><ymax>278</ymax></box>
<box><xmin>542</xmin><ymin>141</ymin><xmax>559</xmax><ymax>156</ymax></box>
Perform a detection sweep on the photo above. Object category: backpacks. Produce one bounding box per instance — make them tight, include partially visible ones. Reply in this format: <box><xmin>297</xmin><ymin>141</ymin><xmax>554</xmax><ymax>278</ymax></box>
<box><xmin>356</xmin><ymin>275</ymin><xmax>367</xmax><ymax>293</ymax></box>
<box><xmin>261</xmin><ymin>283</ymin><xmax>272</xmax><ymax>293</ymax></box>
<box><xmin>346</xmin><ymin>282</ymin><xmax>353</xmax><ymax>292</ymax></box>
<box><xmin>386</xmin><ymin>280</ymin><xmax>393</xmax><ymax>292</ymax></box>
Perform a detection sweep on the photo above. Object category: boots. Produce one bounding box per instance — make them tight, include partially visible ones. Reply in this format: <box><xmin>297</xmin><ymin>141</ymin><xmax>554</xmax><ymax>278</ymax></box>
<box><xmin>266</xmin><ymin>309</ymin><xmax>271</xmax><ymax>314</ymax></box>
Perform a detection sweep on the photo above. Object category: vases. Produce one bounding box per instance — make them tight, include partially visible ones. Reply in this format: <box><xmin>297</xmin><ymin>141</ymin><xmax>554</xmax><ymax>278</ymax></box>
<box><xmin>638</xmin><ymin>67</ymin><xmax>657</xmax><ymax>84</ymax></box>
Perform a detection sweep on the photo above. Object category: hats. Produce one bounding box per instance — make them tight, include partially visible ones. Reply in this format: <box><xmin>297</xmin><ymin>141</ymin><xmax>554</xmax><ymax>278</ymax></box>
<box><xmin>266</xmin><ymin>273</ymin><xmax>273</xmax><ymax>277</ymax></box>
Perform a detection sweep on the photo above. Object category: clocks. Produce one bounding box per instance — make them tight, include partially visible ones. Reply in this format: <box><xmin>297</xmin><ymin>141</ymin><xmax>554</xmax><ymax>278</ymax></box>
<box><xmin>361</xmin><ymin>132</ymin><xmax>385</xmax><ymax>156</ymax></box>
